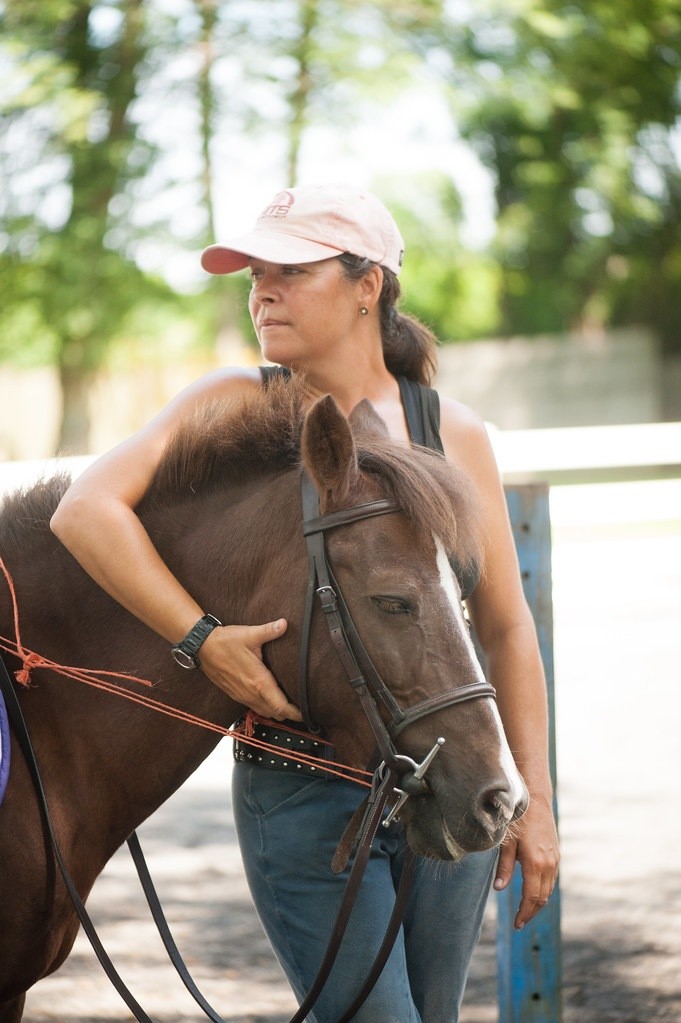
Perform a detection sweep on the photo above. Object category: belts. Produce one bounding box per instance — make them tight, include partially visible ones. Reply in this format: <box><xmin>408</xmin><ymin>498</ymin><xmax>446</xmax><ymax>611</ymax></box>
<box><xmin>235</xmin><ymin>717</ymin><xmax>344</xmax><ymax>779</ymax></box>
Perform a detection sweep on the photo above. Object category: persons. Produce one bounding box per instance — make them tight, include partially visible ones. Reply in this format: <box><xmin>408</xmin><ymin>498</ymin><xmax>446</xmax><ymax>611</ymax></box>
<box><xmin>51</xmin><ymin>174</ymin><xmax>559</xmax><ymax>1022</ymax></box>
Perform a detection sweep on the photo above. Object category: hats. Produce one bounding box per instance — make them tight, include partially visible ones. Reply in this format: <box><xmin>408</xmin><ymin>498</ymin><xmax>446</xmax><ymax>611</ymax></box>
<box><xmin>200</xmin><ymin>184</ymin><xmax>404</xmax><ymax>276</ymax></box>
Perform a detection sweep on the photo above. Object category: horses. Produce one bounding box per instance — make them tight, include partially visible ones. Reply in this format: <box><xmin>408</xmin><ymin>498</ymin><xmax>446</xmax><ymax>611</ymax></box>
<box><xmin>0</xmin><ymin>392</ymin><xmax>530</xmax><ymax>1022</ymax></box>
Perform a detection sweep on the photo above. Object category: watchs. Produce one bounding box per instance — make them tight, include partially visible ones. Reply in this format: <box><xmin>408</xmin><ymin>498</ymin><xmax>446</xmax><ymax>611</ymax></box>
<box><xmin>171</xmin><ymin>613</ymin><xmax>221</xmax><ymax>671</ymax></box>
